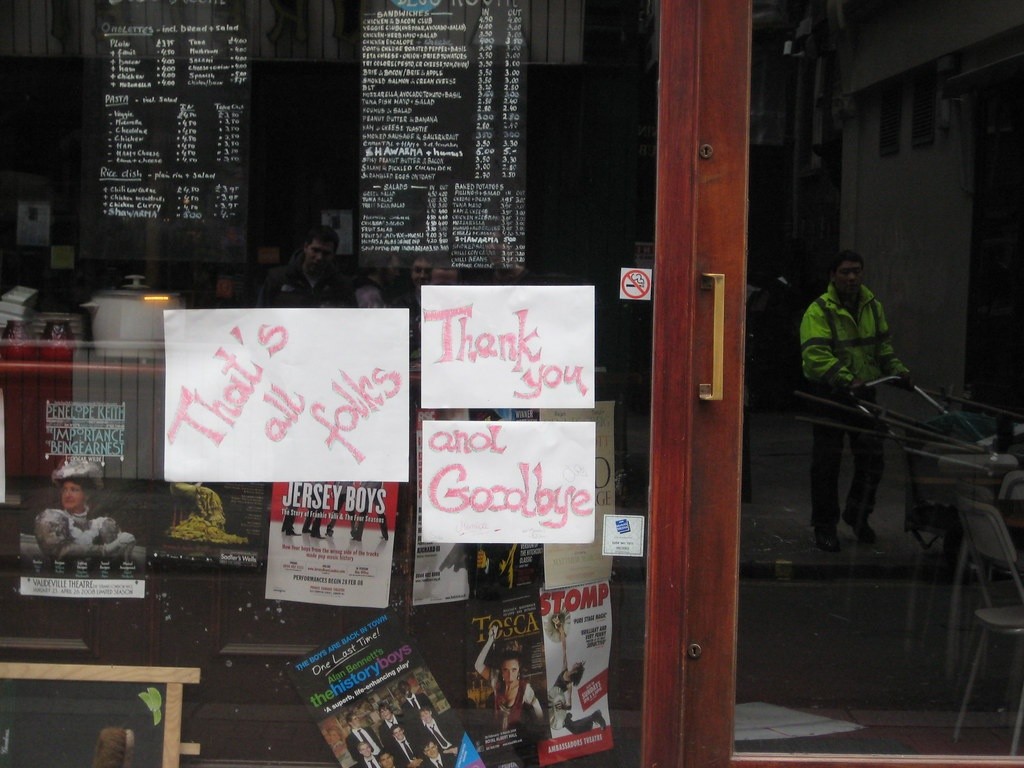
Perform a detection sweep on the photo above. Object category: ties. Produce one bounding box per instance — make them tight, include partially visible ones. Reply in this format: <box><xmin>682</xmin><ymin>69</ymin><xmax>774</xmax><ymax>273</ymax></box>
<box><xmin>368</xmin><ymin>759</ymin><xmax>377</xmax><ymax>768</ymax></box>
<box><xmin>357</xmin><ymin>729</ymin><xmax>374</xmax><ymax>752</ymax></box>
<box><xmin>436</xmin><ymin>760</ymin><xmax>443</xmax><ymax>768</ymax></box>
<box><xmin>430</xmin><ymin>725</ymin><xmax>447</xmax><ymax>747</ymax></box>
<box><xmin>411</xmin><ymin>698</ymin><xmax>418</xmax><ymax>710</ymax></box>
<box><xmin>402</xmin><ymin>740</ymin><xmax>414</xmax><ymax>760</ymax></box>
<box><xmin>389</xmin><ymin>719</ymin><xmax>394</xmax><ymax>725</ymax></box>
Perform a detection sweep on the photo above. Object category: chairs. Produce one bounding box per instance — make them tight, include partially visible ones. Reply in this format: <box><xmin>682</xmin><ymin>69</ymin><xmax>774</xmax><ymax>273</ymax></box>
<box><xmin>900</xmin><ymin>445</ymin><xmax>1024</xmax><ymax>758</ymax></box>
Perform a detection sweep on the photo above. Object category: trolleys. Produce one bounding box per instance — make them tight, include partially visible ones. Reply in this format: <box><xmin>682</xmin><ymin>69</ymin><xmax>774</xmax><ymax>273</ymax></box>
<box><xmin>851</xmin><ymin>375</ymin><xmax>1024</xmax><ymax>598</ymax></box>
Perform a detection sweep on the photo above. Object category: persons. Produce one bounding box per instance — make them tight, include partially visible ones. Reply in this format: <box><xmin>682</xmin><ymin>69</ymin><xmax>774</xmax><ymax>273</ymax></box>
<box><xmin>800</xmin><ymin>253</ymin><xmax>914</xmax><ymax>549</ymax></box>
<box><xmin>550</xmin><ymin>617</ymin><xmax>609</xmax><ymax>736</ymax></box>
<box><xmin>35</xmin><ymin>456</ymin><xmax>134</xmax><ymax>577</ymax></box>
<box><xmin>347</xmin><ymin>681</ymin><xmax>462</xmax><ymax>768</ymax></box>
<box><xmin>279</xmin><ymin>482</ymin><xmax>391</xmax><ymax>542</ymax></box>
<box><xmin>255</xmin><ymin>228</ymin><xmax>543</xmax><ymax>359</ymax></box>
<box><xmin>476</xmin><ymin>627</ymin><xmax>547</xmax><ymax>746</ymax></box>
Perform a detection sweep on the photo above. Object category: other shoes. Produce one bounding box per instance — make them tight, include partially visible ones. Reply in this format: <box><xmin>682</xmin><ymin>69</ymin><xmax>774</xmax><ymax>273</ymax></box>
<box><xmin>302</xmin><ymin>528</ymin><xmax>312</xmax><ymax>533</ymax></box>
<box><xmin>843</xmin><ymin>506</ymin><xmax>876</xmax><ymax>543</ymax></box>
<box><xmin>814</xmin><ymin>526</ymin><xmax>840</xmax><ymax>551</ymax></box>
<box><xmin>381</xmin><ymin>534</ymin><xmax>388</xmax><ymax>540</ymax></box>
<box><xmin>327</xmin><ymin>526</ymin><xmax>333</xmax><ymax>537</ymax></box>
<box><xmin>310</xmin><ymin>533</ymin><xmax>324</xmax><ymax>539</ymax></box>
<box><xmin>351</xmin><ymin>529</ymin><xmax>361</xmax><ymax>541</ymax></box>
<box><xmin>282</xmin><ymin>526</ymin><xmax>301</xmax><ymax>536</ymax></box>
<box><xmin>593</xmin><ymin>710</ymin><xmax>606</xmax><ymax>730</ymax></box>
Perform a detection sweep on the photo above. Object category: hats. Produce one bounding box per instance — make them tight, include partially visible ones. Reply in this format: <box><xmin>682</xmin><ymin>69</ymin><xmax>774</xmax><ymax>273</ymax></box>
<box><xmin>51</xmin><ymin>456</ymin><xmax>103</xmax><ymax>491</ymax></box>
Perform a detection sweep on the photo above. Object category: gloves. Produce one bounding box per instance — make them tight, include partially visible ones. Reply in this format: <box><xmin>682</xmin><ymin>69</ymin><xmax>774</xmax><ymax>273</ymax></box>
<box><xmin>474</xmin><ymin>626</ymin><xmax>498</xmax><ymax>674</ymax></box>
<box><xmin>849</xmin><ymin>379</ymin><xmax>876</xmax><ymax>399</ymax></box>
<box><xmin>893</xmin><ymin>373</ymin><xmax>914</xmax><ymax>391</ymax></box>
<box><xmin>102</xmin><ymin>532</ymin><xmax>136</xmax><ymax>557</ymax></box>
